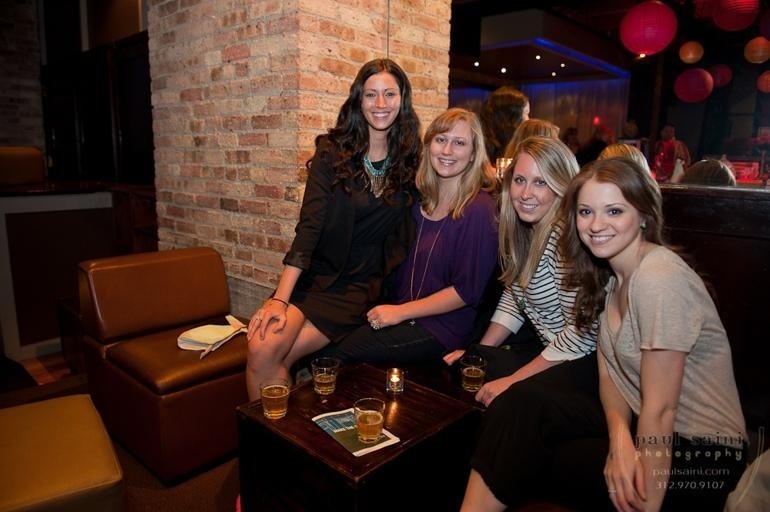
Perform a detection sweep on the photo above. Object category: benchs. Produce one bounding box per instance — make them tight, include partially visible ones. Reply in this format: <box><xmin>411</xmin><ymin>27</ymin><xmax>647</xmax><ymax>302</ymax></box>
<box><xmin>369</xmin><ymin>170</ymin><xmax>770</xmax><ymax>512</ymax></box>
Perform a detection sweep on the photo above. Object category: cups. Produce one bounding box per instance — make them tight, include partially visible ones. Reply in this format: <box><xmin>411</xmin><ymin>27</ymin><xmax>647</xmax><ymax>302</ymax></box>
<box><xmin>386</xmin><ymin>367</ymin><xmax>405</xmax><ymax>391</ymax></box>
<box><xmin>458</xmin><ymin>353</ymin><xmax>485</xmax><ymax>392</ymax></box>
<box><xmin>259</xmin><ymin>378</ymin><xmax>288</xmax><ymax>419</ymax></box>
<box><xmin>352</xmin><ymin>397</ymin><xmax>386</xmax><ymax>443</ymax></box>
<box><xmin>312</xmin><ymin>357</ymin><xmax>339</xmax><ymax>395</ymax></box>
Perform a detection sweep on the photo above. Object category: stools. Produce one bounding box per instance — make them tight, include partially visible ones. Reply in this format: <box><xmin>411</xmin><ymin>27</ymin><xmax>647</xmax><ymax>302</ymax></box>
<box><xmin>1</xmin><ymin>394</ymin><xmax>127</xmax><ymax>510</ymax></box>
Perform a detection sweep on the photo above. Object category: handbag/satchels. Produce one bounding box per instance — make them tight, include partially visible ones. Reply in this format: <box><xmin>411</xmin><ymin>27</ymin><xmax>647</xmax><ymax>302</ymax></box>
<box><xmin>176</xmin><ymin>313</ymin><xmax>249</xmax><ymax>360</ymax></box>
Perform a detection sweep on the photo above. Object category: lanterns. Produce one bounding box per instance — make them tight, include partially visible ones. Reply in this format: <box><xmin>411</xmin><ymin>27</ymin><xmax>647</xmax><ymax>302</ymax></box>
<box><xmin>621</xmin><ymin>1</ymin><xmax>770</xmax><ymax>103</ymax></box>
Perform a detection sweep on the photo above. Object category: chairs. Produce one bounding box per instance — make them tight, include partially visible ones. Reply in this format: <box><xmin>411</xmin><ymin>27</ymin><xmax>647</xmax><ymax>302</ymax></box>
<box><xmin>76</xmin><ymin>246</ymin><xmax>272</xmax><ymax>486</ymax></box>
<box><xmin>1</xmin><ymin>146</ymin><xmax>54</xmax><ymax>193</ymax></box>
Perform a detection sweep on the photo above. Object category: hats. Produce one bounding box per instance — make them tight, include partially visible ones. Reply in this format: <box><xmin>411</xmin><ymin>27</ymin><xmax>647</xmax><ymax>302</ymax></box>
<box><xmin>564</xmin><ymin>127</ymin><xmax>576</xmax><ymax>137</ymax></box>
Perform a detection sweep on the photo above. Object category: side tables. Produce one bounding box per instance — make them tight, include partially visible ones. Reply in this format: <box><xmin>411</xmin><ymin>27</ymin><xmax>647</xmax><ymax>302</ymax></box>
<box><xmin>231</xmin><ymin>363</ymin><xmax>487</xmax><ymax>511</ymax></box>
